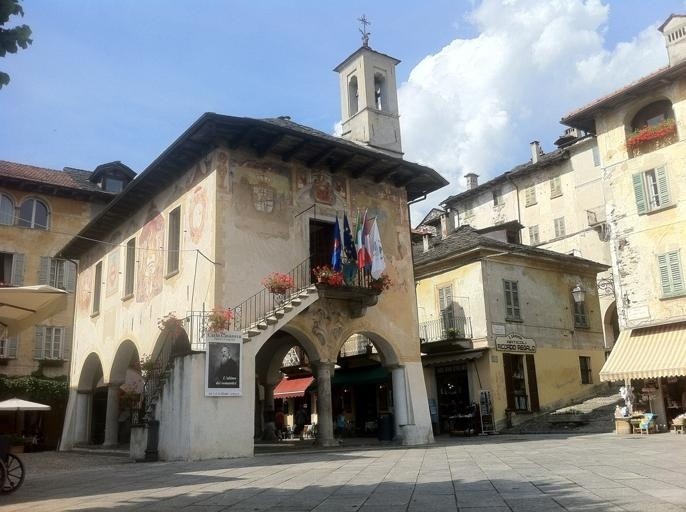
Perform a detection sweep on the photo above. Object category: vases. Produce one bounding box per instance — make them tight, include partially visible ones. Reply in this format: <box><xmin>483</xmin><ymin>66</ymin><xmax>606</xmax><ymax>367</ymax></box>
<box><xmin>269</xmin><ymin>287</ymin><xmax>286</xmax><ymax>293</ymax></box>
<box><xmin>318</xmin><ymin>277</ymin><xmax>327</xmax><ymax>282</ymax></box>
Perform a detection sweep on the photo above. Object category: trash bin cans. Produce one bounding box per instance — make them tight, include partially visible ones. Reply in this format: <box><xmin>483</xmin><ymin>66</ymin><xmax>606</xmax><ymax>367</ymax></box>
<box><xmin>379</xmin><ymin>415</ymin><xmax>392</xmax><ymax>440</ymax></box>
<box><xmin>130</xmin><ymin>420</ymin><xmax>159</xmax><ymax>464</ymax></box>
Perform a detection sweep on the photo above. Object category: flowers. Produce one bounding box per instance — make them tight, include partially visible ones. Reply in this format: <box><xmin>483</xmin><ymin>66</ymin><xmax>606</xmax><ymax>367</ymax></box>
<box><xmin>260</xmin><ymin>271</ymin><xmax>295</xmax><ymax>291</ymax></box>
<box><xmin>156</xmin><ymin>310</ymin><xmax>184</xmax><ymax>337</ymax></box>
<box><xmin>312</xmin><ymin>265</ymin><xmax>333</xmax><ymax>278</ymax></box>
<box><xmin>205</xmin><ymin>305</ymin><xmax>233</xmax><ymax>335</ymax></box>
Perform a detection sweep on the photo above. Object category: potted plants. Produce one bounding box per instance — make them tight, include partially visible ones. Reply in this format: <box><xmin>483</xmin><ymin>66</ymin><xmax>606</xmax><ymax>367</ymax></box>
<box><xmin>448</xmin><ymin>327</ymin><xmax>461</xmax><ymax>336</ymax></box>
<box><xmin>369</xmin><ymin>274</ymin><xmax>391</xmax><ymax>290</ymax></box>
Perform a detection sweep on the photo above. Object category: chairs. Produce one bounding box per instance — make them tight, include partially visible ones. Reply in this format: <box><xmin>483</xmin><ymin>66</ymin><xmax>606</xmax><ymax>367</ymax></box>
<box><xmin>640</xmin><ymin>414</ymin><xmax>659</xmax><ymax>435</ymax></box>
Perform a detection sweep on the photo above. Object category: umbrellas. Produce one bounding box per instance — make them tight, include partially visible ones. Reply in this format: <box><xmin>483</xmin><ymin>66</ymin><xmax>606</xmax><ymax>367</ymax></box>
<box><xmin>0</xmin><ymin>397</ymin><xmax>52</xmax><ymax>412</ymax></box>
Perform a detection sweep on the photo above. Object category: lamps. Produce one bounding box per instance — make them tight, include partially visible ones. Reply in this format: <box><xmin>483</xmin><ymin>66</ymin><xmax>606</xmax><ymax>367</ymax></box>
<box><xmin>571</xmin><ymin>283</ymin><xmax>586</xmax><ymax>326</ymax></box>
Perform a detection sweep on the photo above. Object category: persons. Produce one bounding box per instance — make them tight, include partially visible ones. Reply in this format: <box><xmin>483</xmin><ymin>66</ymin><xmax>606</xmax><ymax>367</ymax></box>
<box><xmin>264</xmin><ymin>404</ymin><xmax>276</xmax><ymax>421</ymax></box>
<box><xmin>213</xmin><ymin>345</ymin><xmax>238</xmax><ymax>387</ymax></box>
<box><xmin>274</xmin><ymin>408</ymin><xmax>285</xmax><ymax>433</ymax></box>
<box><xmin>295</xmin><ymin>404</ymin><xmax>308</xmax><ymax>437</ymax></box>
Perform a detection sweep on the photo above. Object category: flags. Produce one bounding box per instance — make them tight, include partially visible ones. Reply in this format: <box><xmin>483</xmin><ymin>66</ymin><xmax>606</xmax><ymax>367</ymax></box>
<box><xmin>331</xmin><ymin>210</ymin><xmax>387</xmax><ymax>287</ymax></box>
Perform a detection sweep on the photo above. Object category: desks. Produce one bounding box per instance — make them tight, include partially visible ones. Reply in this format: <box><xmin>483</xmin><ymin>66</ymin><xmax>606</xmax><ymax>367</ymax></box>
<box><xmin>448</xmin><ymin>416</ymin><xmax>478</xmax><ymax>437</ymax></box>
<box><xmin>630</xmin><ymin>421</ymin><xmax>640</xmax><ymax>433</ymax></box>
<box><xmin>671</xmin><ymin>423</ymin><xmax>686</xmax><ymax>434</ymax></box>
